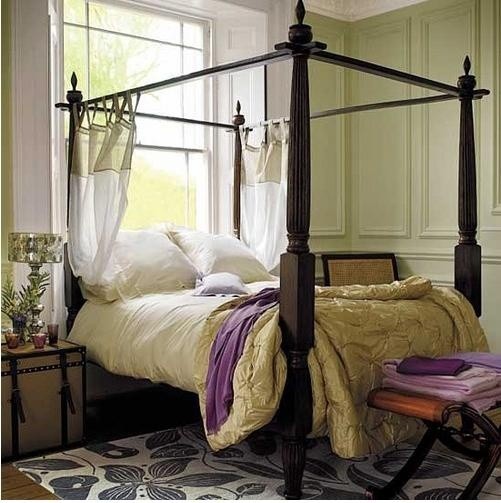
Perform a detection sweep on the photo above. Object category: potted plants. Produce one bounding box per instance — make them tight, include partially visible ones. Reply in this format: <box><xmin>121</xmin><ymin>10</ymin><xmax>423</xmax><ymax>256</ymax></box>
<box><xmin>1</xmin><ymin>271</ymin><xmax>51</xmax><ymax>345</ymax></box>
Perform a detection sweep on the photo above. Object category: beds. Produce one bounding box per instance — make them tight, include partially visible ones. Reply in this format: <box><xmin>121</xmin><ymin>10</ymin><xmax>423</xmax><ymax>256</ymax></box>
<box><xmin>53</xmin><ymin>1</ymin><xmax>490</xmax><ymax>500</ymax></box>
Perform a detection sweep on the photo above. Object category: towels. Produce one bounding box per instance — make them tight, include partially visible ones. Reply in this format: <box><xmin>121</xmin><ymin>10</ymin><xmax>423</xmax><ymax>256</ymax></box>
<box><xmin>396</xmin><ymin>355</ymin><xmax>472</xmax><ymax>376</ymax></box>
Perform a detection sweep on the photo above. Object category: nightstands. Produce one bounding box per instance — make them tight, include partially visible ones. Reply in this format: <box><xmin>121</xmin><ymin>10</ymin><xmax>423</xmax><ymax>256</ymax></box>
<box><xmin>1</xmin><ymin>336</ymin><xmax>88</xmax><ymax>463</ymax></box>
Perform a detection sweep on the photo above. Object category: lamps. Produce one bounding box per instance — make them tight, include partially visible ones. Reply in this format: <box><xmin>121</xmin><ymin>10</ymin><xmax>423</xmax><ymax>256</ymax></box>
<box><xmin>8</xmin><ymin>231</ymin><xmax>64</xmax><ymax>334</ymax></box>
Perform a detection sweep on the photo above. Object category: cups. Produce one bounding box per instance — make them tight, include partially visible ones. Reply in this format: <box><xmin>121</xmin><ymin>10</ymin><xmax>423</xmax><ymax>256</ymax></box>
<box><xmin>31</xmin><ymin>334</ymin><xmax>45</xmax><ymax>348</ymax></box>
<box><xmin>5</xmin><ymin>331</ymin><xmax>21</xmax><ymax>347</ymax></box>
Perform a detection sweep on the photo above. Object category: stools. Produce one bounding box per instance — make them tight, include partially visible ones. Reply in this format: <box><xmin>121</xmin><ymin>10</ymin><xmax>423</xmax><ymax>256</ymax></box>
<box><xmin>361</xmin><ymin>386</ymin><xmax>501</xmax><ymax>500</ymax></box>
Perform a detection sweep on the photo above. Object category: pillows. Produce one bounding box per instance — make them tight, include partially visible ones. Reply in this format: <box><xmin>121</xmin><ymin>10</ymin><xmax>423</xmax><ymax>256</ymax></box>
<box><xmin>193</xmin><ymin>271</ymin><xmax>253</xmax><ymax>298</ymax></box>
<box><xmin>84</xmin><ymin>281</ymin><xmax>120</xmax><ymax>302</ymax></box>
<box><xmin>105</xmin><ymin>227</ymin><xmax>201</xmax><ymax>291</ymax></box>
<box><xmin>168</xmin><ymin>228</ymin><xmax>277</xmax><ymax>283</ymax></box>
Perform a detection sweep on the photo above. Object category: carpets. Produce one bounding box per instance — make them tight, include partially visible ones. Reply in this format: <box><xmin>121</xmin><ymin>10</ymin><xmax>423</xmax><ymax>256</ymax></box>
<box><xmin>12</xmin><ymin>420</ymin><xmax>501</xmax><ymax>500</ymax></box>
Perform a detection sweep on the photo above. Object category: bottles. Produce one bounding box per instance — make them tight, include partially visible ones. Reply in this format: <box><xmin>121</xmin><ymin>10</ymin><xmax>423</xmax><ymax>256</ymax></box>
<box><xmin>48</xmin><ymin>307</ymin><xmax>58</xmax><ymax>344</ymax></box>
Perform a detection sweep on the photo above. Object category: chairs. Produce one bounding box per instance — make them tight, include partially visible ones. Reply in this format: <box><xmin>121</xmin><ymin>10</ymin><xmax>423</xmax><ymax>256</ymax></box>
<box><xmin>320</xmin><ymin>252</ymin><xmax>400</xmax><ymax>287</ymax></box>
